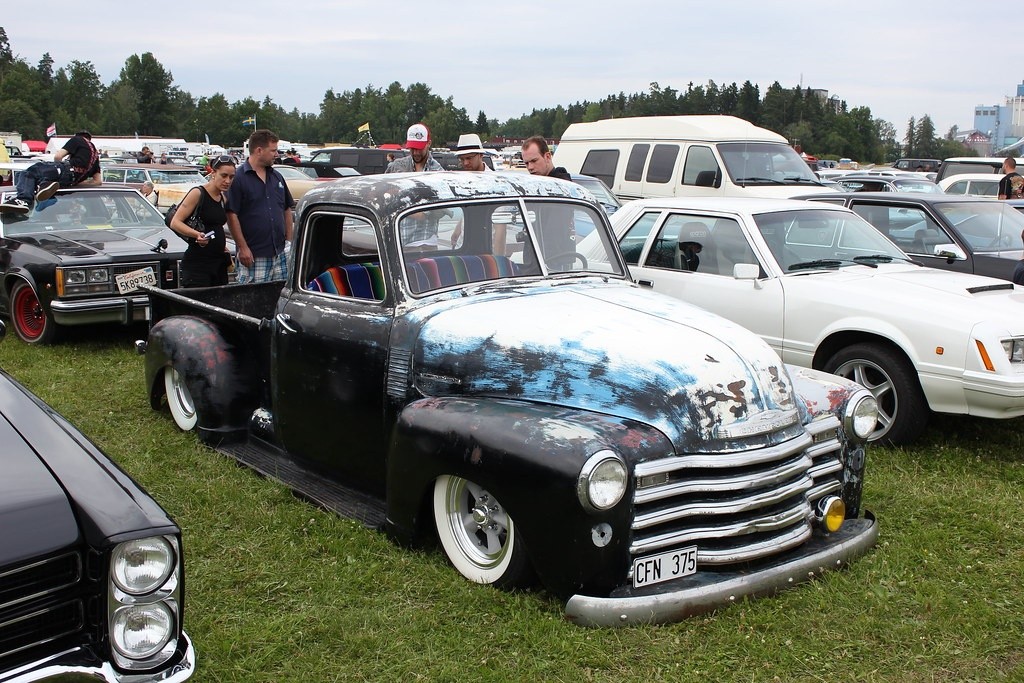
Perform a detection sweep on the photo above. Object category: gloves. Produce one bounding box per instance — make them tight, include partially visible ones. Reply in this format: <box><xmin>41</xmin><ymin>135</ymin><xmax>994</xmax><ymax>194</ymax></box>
<box><xmin>283</xmin><ymin>240</ymin><xmax>292</xmax><ymax>262</ymax></box>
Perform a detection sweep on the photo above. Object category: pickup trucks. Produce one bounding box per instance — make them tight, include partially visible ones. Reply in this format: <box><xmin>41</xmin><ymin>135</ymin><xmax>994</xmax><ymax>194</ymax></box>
<box><xmin>137</xmin><ymin>171</ymin><xmax>879</xmax><ymax>629</ymax></box>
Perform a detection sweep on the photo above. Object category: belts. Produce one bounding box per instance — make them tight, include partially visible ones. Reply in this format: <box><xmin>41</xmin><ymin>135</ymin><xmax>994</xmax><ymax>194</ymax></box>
<box><xmin>67</xmin><ymin>162</ymin><xmax>76</xmax><ymax>183</ymax></box>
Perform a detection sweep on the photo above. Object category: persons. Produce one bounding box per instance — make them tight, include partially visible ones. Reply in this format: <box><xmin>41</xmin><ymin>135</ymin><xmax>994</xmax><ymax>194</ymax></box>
<box><xmin>128</xmin><ymin>147</ymin><xmax>167</xmax><ymax>164</ymax></box>
<box><xmin>46</xmin><ymin>151</ymin><xmax>50</xmax><ymax>154</ymax></box>
<box><xmin>450</xmin><ymin>134</ymin><xmax>507</xmax><ymax>256</ymax></box>
<box><xmin>916</xmin><ymin>164</ymin><xmax>941</xmax><ymax>172</ymax></box>
<box><xmin>0</xmin><ymin>138</ymin><xmax>9</xmax><ymax>175</ymax></box>
<box><xmin>0</xmin><ymin>130</ymin><xmax>102</xmax><ymax>212</ymax></box>
<box><xmin>273</xmin><ymin>150</ymin><xmax>301</xmax><ymax>165</ymax></box>
<box><xmin>821</xmin><ymin>161</ymin><xmax>828</xmax><ymax>168</ymax></box>
<box><xmin>522</xmin><ymin>135</ymin><xmax>576</xmax><ymax>270</ymax></box>
<box><xmin>169</xmin><ymin>155</ymin><xmax>236</xmax><ymax>289</ymax></box>
<box><xmin>829</xmin><ymin>162</ymin><xmax>834</xmax><ymax>168</ymax></box>
<box><xmin>384</xmin><ymin>123</ymin><xmax>448</xmax><ymax>249</ymax></box>
<box><xmin>387</xmin><ymin>153</ymin><xmax>395</xmax><ymax>167</ymax></box>
<box><xmin>133</xmin><ymin>181</ymin><xmax>157</xmax><ymax>217</ymax></box>
<box><xmin>199</xmin><ymin>152</ymin><xmax>239</xmax><ymax>175</ymax></box>
<box><xmin>98</xmin><ymin>149</ymin><xmax>110</xmax><ymax>158</ymax></box>
<box><xmin>223</xmin><ymin>130</ymin><xmax>295</xmax><ymax>284</ymax></box>
<box><xmin>998</xmin><ymin>158</ymin><xmax>1024</xmax><ymax>200</ymax></box>
<box><xmin>1013</xmin><ymin>230</ymin><xmax>1024</xmax><ymax>286</ymax></box>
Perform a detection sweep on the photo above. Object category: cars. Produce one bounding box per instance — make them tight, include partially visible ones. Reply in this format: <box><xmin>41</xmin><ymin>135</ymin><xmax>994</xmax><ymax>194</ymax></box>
<box><xmin>0</xmin><ymin>184</ymin><xmax>189</xmax><ymax>346</ymax></box>
<box><xmin>935</xmin><ymin>156</ymin><xmax>1024</xmax><ymax>185</ymax></box>
<box><xmin>568</xmin><ymin>174</ymin><xmax>622</xmax><ymax>240</ymax></box>
<box><xmin>780</xmin><ymin>192</ymin><xmax>1024</xmax><ymax>286</ymax></box>
<box><xmin>816</xmin><ymin>167</ymin><xmax>929</xmax><ymax>180</ymax></box>
<box><xmin>0</xmin><ymin>145</ymin><xmax>334</xmax><ymax>222</ymax></box>
<box><xmin>0</xmin><ymin>368</ymin><xmax>195</xmax><ymax>683</ymax></box>
<box><xmin>511</xmin><ymin>197</ymin><xmax>1024</xmax><ymax>451</ymax></box>
<box><xmin>829</xmin><ymin>176</ymin><xmax>944</xmax><ymax>193</ymax></box>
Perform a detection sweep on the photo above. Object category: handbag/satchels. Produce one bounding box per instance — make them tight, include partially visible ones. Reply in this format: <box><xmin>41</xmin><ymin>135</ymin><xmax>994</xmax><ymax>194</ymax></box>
<box><xmin>164</xmin><ymin>186</ymin><xmax>204</xmax><ymax>245</ymax></box>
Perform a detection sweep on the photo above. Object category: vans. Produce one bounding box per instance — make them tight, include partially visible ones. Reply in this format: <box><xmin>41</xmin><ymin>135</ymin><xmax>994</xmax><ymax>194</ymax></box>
<box><xmin>290</xmin><ymin>148</ymin><xmax>410</xmax><ymax>176</ymax></box>
<box><xmin>551</xmin><ymin>114</ymin><xmax>844</xmax><ymax>208</ymax></box>
<box><xmin>938</xmin><ymin>173</ymin><xmax>1024</xmax><ymax>197</ymax></box>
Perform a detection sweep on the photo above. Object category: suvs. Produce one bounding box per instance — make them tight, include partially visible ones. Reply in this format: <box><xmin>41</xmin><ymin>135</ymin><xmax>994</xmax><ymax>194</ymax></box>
<box><xmin>894</xmin><ymin>158</ymin><xmax>942</xmax><ymax>172</ymax></box>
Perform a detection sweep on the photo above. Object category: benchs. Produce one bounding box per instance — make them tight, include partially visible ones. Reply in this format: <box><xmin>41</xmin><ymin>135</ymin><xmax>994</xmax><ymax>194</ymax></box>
<box><xmin>309</xmin><ymin>254</ymin><xmax>520</xmax><ymax>301</ymax></box>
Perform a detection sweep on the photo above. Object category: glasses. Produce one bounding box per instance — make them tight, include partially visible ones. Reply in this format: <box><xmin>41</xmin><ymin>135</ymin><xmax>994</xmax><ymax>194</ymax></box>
<box><xmin>213</xmin><ymin>155</ymin><xmax>238</xmax><ymax>168</ymax></box>
<box><xmin>457</xmin><ymin>154</ymin><xmax>479</xmax><ymax>160</ymax></box>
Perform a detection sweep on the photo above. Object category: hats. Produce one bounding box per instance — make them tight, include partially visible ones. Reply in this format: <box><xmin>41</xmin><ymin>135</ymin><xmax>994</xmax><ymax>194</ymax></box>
<box><xmin>75</xmin><ymin>129</ymin><xmax>91</xmax><ymax>138</ymax></box>
<box><xmin>406</xmin><ymin>123</ymin><xmax>431</xmax><ymax>149</ymax></box>
<box><xmin>453</xmin><ymin>134</ymin><xmax>486</xmax><ymax>155</ymax></box>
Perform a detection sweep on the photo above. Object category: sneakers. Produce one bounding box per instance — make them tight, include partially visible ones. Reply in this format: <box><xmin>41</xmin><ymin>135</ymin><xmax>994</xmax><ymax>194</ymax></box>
<box><xmin>0</xmin><ymin>198</ymin><xmax>30</xmax><ymax>213</ymax></box>
<box><xmin>0</xmin><ymin>212</ymin><xmax>29</xmax><ymax>224</ymax></box>
<box><xmin>34</xmin><ymin>196</ymin><xmax>57</xmax><ymax>212</ymax></box>
<box><xmin>36</xmin><ymin>180</ymin><xmax>60</xmax><ymax>202</ymax></box>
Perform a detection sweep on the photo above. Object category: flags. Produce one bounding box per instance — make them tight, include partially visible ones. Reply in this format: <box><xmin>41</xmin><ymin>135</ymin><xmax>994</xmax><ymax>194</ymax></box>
<box><xmin>47</xmin><ymin>123</ymin><xmax>55</xmax><ymax>137</ymax></box>
<box><xmin>358</xmin><ymin>123</ymin><xmax>369</xmax><ymax>132</ymax></box>
<box><xmin>242</xmin><ymin>114</ymin><xmax>255</xmax><ymax>126</ymax></box>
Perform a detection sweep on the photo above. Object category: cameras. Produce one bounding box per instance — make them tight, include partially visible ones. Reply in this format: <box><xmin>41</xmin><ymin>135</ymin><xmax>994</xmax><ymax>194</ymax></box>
<box><xmin>205</xmin><ymin>231</ymin><xmax>216</xmax><ymax>240</ymax></box>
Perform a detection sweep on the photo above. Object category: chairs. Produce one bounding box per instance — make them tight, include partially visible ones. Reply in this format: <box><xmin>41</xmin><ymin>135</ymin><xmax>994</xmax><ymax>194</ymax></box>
<box><xmin>153</xmin><ymin>190</ymin><xmax>159</xmax><ymax>210</ymax></box>
<box><xmin>912</xmin><ymin>228</ymin><xmax>952</xmax><ymax>256</ymax></box>
<box><xmin>676</xmin><ymin>222</ymin><xmax>720</xmax><ymax>275</ymax></box>
